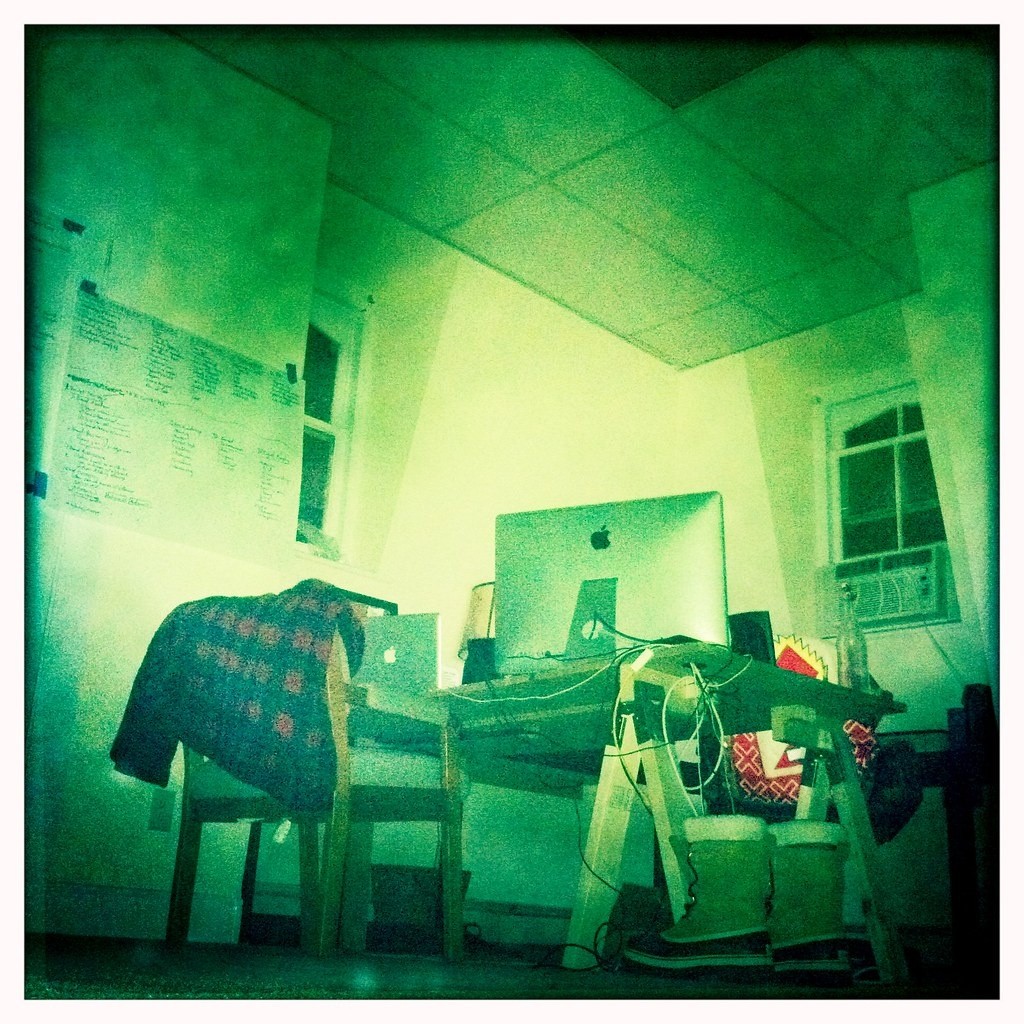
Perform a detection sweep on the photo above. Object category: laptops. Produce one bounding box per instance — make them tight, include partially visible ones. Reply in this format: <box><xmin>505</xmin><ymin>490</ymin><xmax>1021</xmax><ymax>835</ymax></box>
<box><xmin>494</xmin><ymin>491</ymin><xmax>733</xmax><ymax>677</ymax></box>
<box><xmin>351</xmin><ymin>612</ymin><xmax>443</xmax><ymax>691</ymax></box>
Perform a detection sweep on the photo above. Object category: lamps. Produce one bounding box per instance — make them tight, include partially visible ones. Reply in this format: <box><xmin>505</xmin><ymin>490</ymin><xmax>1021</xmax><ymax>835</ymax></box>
<box><xmin>457</xmin><ymin>582</ymin><xmax>494</xmax><ymax>684</ymax></box>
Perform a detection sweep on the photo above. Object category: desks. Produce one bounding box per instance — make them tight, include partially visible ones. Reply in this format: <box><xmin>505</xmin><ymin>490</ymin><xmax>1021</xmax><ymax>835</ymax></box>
<box><xmin>345</xmin><ymin>642</ymin><xmax>910</xmax><ymax>982</ymax></box>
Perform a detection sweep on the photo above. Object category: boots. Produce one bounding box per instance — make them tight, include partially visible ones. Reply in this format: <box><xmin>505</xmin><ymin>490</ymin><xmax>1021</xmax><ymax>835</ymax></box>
<box><xmin>624</xmin><ymin>812</ymin><xmax>853</xmax><ymax>981</ymax></box>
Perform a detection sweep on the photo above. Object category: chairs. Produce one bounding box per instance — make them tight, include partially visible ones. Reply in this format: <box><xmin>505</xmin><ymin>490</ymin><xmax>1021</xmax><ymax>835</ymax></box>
<box><xmin>163</xmin><ymin>631</ymin><xmax>464</xmax><ymax>963</ymax></box>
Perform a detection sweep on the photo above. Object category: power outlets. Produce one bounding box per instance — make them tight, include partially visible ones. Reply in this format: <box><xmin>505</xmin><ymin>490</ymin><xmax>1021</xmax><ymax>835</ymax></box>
<box><xmin>149</xmin><ymin>788</ymin><xmax>176</xmax><ymax>833</ymax></box>
